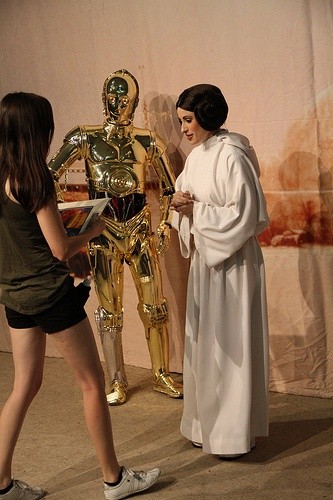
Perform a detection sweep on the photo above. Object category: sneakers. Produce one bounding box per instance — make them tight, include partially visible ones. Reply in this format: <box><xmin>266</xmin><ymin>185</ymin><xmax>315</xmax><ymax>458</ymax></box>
<box><xmin>103</xmin><ymin>465</ymin><xmax>161</xmax><ymax>500</ymax></box>
<box><xmin>0</xmin><ymin>476</ymin><xmax>46</xmax><ymax>500</ymax></box>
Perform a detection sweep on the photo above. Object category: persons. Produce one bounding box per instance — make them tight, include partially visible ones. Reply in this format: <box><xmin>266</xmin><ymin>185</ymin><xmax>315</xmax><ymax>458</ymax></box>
<box><xmin>0</xmin><ymin>91</ymin><xmax>161</xmax><ymax>500</ymax></box>
<box><xmin>47</xmin><ymin>69</ymin><xmax>184</xmax><ymax>406</ymax></box>
<box><xmin>168</xmin><ymin>83</ymin><xmax>270</xmax><ymax>460</ymax></box>
